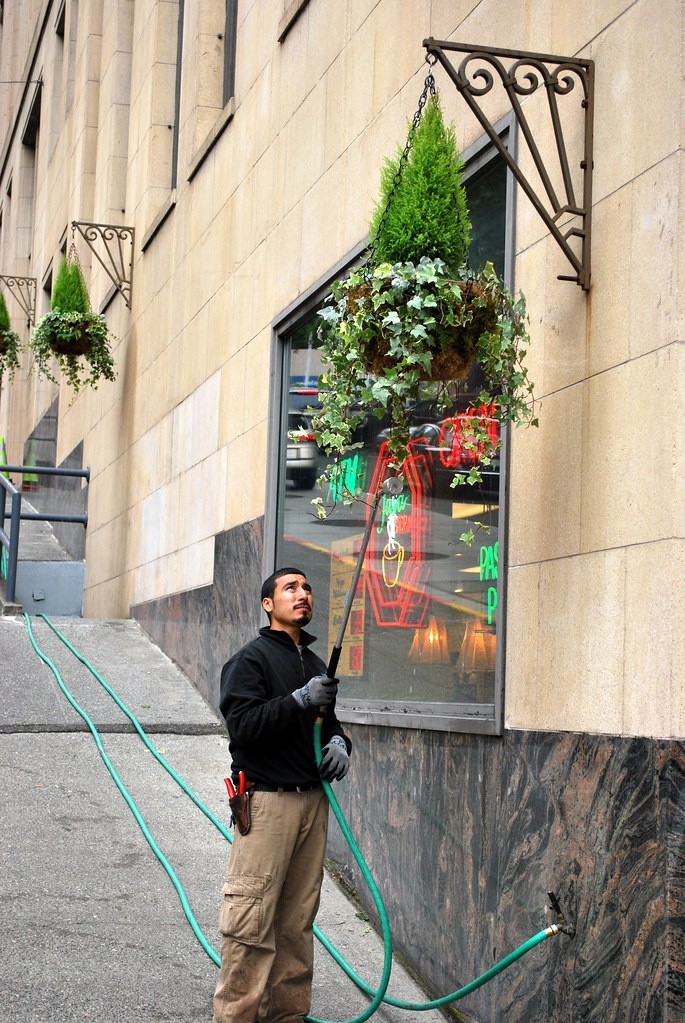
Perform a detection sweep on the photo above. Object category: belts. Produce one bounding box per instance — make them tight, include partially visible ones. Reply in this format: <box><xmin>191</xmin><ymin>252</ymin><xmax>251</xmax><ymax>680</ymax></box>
<box><xmin>252</xmin><ymin>781</ymin><xmax>323</xmax><ymax>794</ymax></box>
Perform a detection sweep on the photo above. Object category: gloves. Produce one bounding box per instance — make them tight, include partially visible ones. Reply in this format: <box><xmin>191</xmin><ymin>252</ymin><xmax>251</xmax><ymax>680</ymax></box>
<box><xmin>318</xmin><ymin>735</ymin><xmax>350</xmax><ymax>782</ymax></box>
<box><xmin>291</xmin><ymin>676</ymin><xmax>340</xmax><ymax>710</ymax></box>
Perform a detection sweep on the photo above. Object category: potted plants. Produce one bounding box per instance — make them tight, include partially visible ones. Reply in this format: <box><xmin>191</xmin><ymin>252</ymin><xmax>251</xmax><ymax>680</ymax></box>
<box><xmin>304</xmin><ymin>87</ymin><xmax>543</xmax><ymax>547</ymax></box>
<box><xmin>0</xmin><ymin>286</ymin><xmax>22</xmax><ymax>383</ymax></box>
<box><xmin>29</xmin><ymin>254</ymin><xmax>119</xmax><ymax>401</ymax></box>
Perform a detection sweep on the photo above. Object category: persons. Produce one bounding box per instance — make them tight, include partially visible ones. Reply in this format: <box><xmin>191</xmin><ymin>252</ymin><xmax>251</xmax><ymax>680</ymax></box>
<box><xmin>212</xmin><ymin>568</ymin><xmax>352</xmax><ymax>1023</ymax></box>
